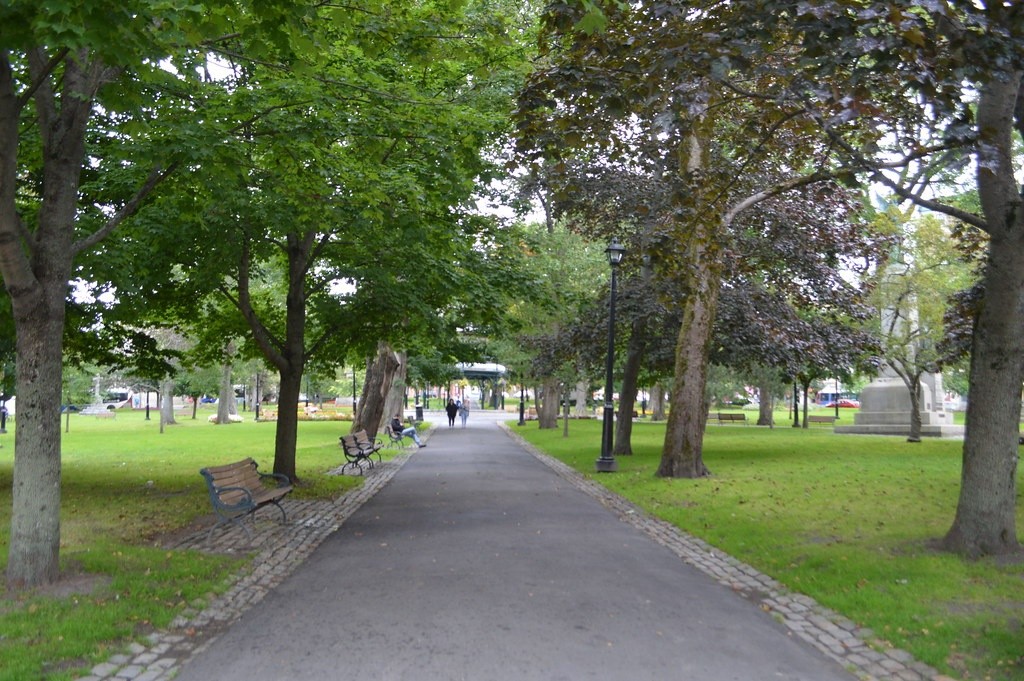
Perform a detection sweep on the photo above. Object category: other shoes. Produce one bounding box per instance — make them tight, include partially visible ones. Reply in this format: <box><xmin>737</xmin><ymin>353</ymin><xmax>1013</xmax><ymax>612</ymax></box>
<box><xmin>419</xmin><ymin>444</ymin><xmax>426</xmax><ymax>448</ymax></box>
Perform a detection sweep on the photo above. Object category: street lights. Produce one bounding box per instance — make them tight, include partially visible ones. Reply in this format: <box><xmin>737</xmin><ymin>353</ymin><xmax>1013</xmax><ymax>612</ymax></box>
<box><xmin>595</xmin><ymin>240</ymin><xmax>626</xmax><ymax>472</ymax></box>
<box><xmin>517</xmin><ymin>342</ymin><xmax>527</xmax><ymax>427</ymax></box>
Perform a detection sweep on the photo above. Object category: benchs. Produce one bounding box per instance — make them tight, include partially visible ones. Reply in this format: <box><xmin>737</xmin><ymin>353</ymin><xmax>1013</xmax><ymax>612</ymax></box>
<box><xmin>615</xmin><ymin>411</ymin><xmax>641</xmax><ymax>423</ymax></box>
<box><xmin>408</xmin><ymin>416</ymin><xmax>422</xmax><ymax>433</ymax></box>
<box><xmin>339</xmin><ymin>434</ymin><xmax>372</xmax><ymax>476</ymax></box>
<box><xmin>200</xmin><ymin>457</ymin><xmax>295</xmax><ymax>549</ymax></box>
<box><xmin>96</xmin><ymin>412</ymin><xmax>116</xmax><ymax>421</ymax></box>
<box><xmin>707</xmin><ymin>413</ymin><xmax>720</xmax><ymax>423</ymax></box>
<box><xmin>718</xmin><ymin>414</ymin><xmax>749</xmax><ymax>427</ymax></box>
<box><xmin>386</xmin><ymin>423</ymin><xmax>404</xmax><ymax>449</ymax></box>
<box><xmin>353</xmin><ymin>428</ymin><xmax>385</xmax><ymax>468</ymax></box>
<box><xmin>808</xmin><ymin>416</ymin><xmax>836</xmax><ymax>428</ymax></box>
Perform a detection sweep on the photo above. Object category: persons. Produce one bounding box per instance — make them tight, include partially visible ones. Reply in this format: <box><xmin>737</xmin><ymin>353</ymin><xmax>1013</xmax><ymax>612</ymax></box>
<box><xmin>391</xmin><ymin>414</ymin><xmax>427</xmax><ymax>448</ymax></box>
<box><xmin>445</xmin><ymin>399</ymin><xmax>469</xmax><ymax>429</ymax></box>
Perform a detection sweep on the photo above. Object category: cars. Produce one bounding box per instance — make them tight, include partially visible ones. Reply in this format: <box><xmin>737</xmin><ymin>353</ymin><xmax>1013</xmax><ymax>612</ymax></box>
<box><xmin>826</xmin><ymin>399</ymin><xmax>859</xmax><ymax>409</ymax></box>
